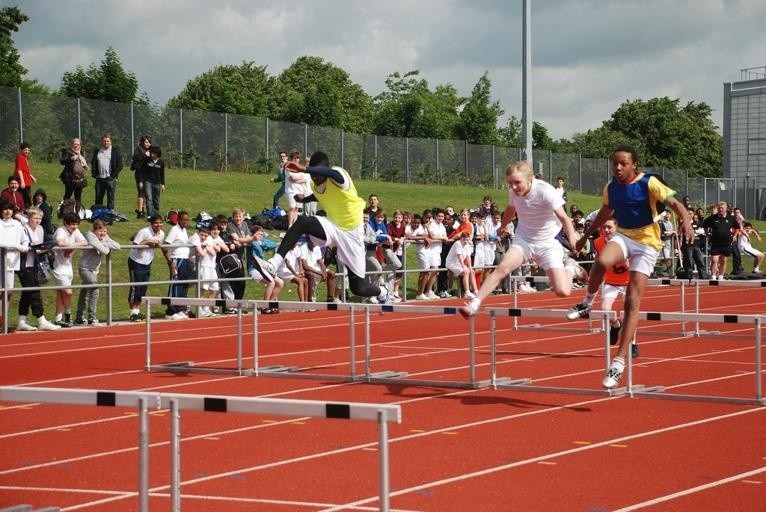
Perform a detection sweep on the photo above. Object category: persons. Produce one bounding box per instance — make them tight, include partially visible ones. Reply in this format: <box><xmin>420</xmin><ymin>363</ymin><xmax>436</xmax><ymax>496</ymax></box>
<box><xmin>14</xmin><ymin>142</ymin><xmax>37</xmax><ymax>206</ymax></box>
<box><xmin>576</xmin><ymin>145</ymin><xmax>695</xmax><ymax>391</ymax></box>
<box><xmin>33</xmin><ymin>189</ymin><xmax>52</xmax><ymax>233</ymax></box>
<box><xmin>593</xmin><ymin>217</ymin><xmax>639</xmax><ymax>358</ymax></box>
<box><xmin>458</xmin><ymin>160</ymin><xmax>588</xmax><ymax>320</ymax></box>
<box><xmin>363</xmin><ymin>194</ymin><xmax>519</xmax><ymax>303</ymax></box>
<box><xmin>252</xmin><ymin>152</ymin><xmax>394</xmax><ymax>305</ymax></box>
<box><xmin>1</xmin><ymin>176</ymin><xmax>25</xmax><ymax>213</ymax></box>
<box><xmin>59</xmin><ymin>138</ymin><xmax>88</xmax><ymax>202</ymax></box>
<box><xmin>270</xmin><ymin>151</ymin><xmax>318</xmax><ymax>228</ymax></box>
<box><xmin>91</xmin><ymin>134</ymin><xmax>123</xmax><ymax>210</ymax></box>
<box><xmin>660</xmin><ymin>196</ymin><xmax>765</xmax><ymax>281</ymax></box>
<box><xmin>554</xmin><ymin>178</ymin><xmax>568</xmax><ymax>213</ymax></box>
<box><xmin>556</xmin><ymin>204</ymin><xmax>601</xmax><ymax>289</ymax></box>
<box><xmin>0</xmin><ymin>202</ymin><xmax>349</xmax><ymax>330</ymax></box>
<box><xmin>130</xmin><ymin>136</ymin><xmax>165</xmax><ymax>221</ymax></box>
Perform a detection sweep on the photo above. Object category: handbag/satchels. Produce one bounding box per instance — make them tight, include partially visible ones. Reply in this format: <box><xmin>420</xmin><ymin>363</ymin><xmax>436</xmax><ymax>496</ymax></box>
<box><xmin>220</xmin><ymin>253</ymin><xmax>242</xmax><ymax>274</ymax></box>
<box><xmin>57</xmin><ymin>199</ymin><xmax>80</xmax><ymax>218</ymax></box>
<box><xmin>74</xmin><ymin>165</ymin><xmax>87</xmax><ymax>189</ymax></box>
<box><xmin>35</xmin><ymin>259</ymin><xmax>52</xmax><ymax>283</ymax></box>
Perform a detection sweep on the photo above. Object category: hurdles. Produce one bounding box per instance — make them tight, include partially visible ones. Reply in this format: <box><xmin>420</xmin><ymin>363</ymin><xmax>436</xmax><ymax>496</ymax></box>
<box><xmin>509</xmin><ymin>275</ymin><xmax>766</xmax><ymax>340</ymax></box>
<box><xmin>0</xmin><ymin>385</ymin><xmax>402</xmax><ymax>512</ymax></box>
<box><xmin>141</xmin><ymin>295</ymin><xmax>766</xmax><ymax>406</ymax></box>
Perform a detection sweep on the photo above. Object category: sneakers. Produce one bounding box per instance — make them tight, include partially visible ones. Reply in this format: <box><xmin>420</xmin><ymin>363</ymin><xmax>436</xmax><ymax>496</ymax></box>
<box><xmin>16</xmin><ymin>308</ymin><xmax>99</xmax><ymax>330</ymax></box>
<box><xmin>251</xmin><ymin>253</ymin><xmax>277</xmax><ymax>282</ymax></box>
<box><xmin>602</xmin><ymin>358</ymin><xmax>623</xmax><ymax>387</ymax></box>
<box><xmin>130</xmin><ymin>304</ymin><xmax>278</xmax><ymax>322</ymax></box>
<box><xmin>566</xmin><ymin>258</ymin><xmax>588</xmax><ymax>283</ymax></box>
<box><xmin>416</xmin><ymin>290</ymin><xmax>481</xmax><ymax>319</ymax></box>
<box><xmin>610</xmin><ymin>320</ymin><xmax>621</xmax><ymax>344</ymax></box>
<box><xmin>632</xmin><ymin>344</ymin><xmax>639</xmax><ymax>357</ymax></box>
<box><xmin>567</xmin><ymin>302</ymin><xmax>592</xmax><ymax>320</ymax></box>
